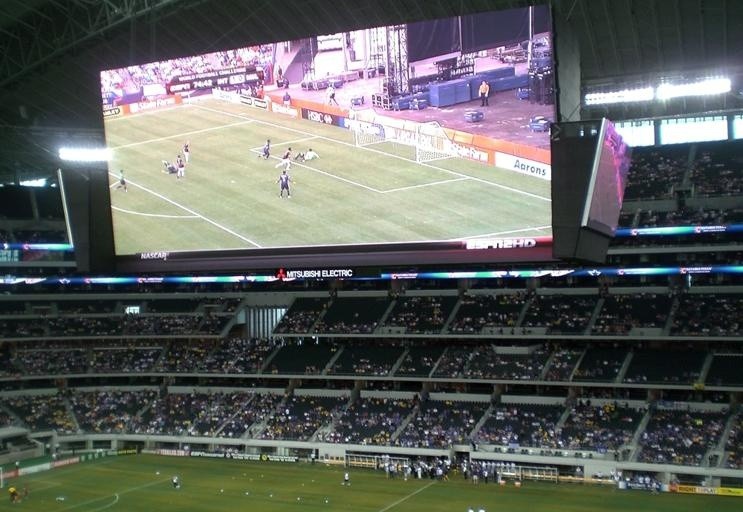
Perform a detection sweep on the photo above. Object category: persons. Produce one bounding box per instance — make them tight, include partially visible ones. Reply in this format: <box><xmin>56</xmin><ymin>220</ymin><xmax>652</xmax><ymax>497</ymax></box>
<box><xmin>607</xmin><ymin>148</ymin><xmax>743</xmax><ymax>269</ymax></box>
<box><xmin>257</xmin><ymin>139</ymin><xmax>271</xmax><ymax>160</ymax></box>
<box><xmin>274</xmin><ymin>147</ymin><xmax>294</xmax><ymax>170</ymax></box>
<box><xmin>181</xmin><ymin>141</ymin><xmax>189</xmax><ymax>164</ymax></box>
<box><xmin>327</xmin><ymin>84</ymin><xmax>340</xmax><ymax>107</ymax></box>
<box><xmin>159</xmin><ymin>159</ymin><xmax>177</xmax><ymax>175</ymax></box>
<box><xmin>8</xmin><ymin>486</ymin><xmax>19</xmax><ymax>503</ymax></box>
<box><xmin>116</xmin><ymin>168</ymin><xmax>128</xmax><ymax>192</ymax></box>
<box><xmin>275</xmin><ymin>170</ymin><xmax>296</xmax><ymax>199</ymax></box>
<box><xmin>295</xmin><ymin>148</ymin><xmax>321</xmax><ymax>162</ymax></box>
<box><xmin>479</xmin><ymin>80</ymin><xmax>489</xmax><ymax>105</ymax></box>
<box><xmin>175</xmin><ymin>154</ymin><xmax>185</xmax><ymax>178</ymax></box>
<box><xmin>100</xmin><ymin>39</ymin><xmax>292</xmax><ymax>109</ymax></box>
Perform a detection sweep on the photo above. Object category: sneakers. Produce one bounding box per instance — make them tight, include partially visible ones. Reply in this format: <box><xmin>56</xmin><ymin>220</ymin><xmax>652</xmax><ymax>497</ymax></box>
<box><xmin>279</xmin><ymin>195</ymin><xmax>291</xmax><ymax>199</ymax></box>
